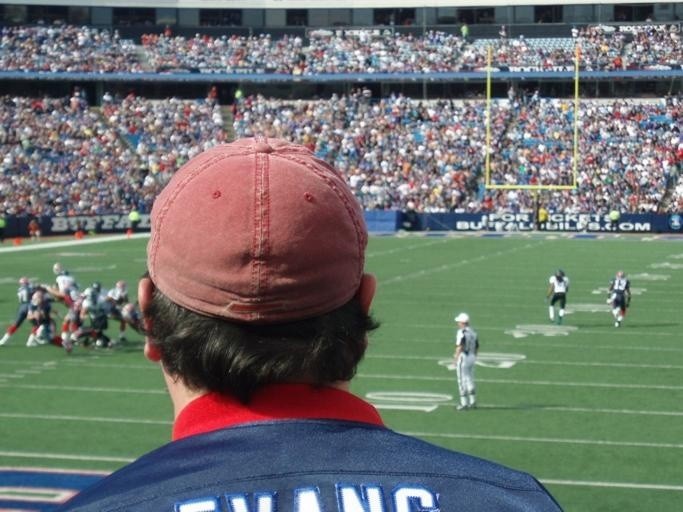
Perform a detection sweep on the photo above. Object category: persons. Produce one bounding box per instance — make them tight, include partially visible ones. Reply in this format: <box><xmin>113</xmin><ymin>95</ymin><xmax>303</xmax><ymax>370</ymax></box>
<box><xmin>543</xmin><ymin>268</ymin><xmax>570</xmax><ymax>326</ymax></box>
<box><xmin>0</xmin><ymin>260</ymin><xmax>144</xmax><ymax>353</ymax></box>
<box><xmin>605</xmin><ymin>270</ymin><xmax>631</xmax><ymax>328</ymax></box>
<box><xmin>52</xmin><ymin>132</ymin><xmax>565</xmax><ymax>512</ymax></box>
<box><xmin>451</xmin><ymin>310</ymin><xmax>481</xmax><ymax>412</ymax></box>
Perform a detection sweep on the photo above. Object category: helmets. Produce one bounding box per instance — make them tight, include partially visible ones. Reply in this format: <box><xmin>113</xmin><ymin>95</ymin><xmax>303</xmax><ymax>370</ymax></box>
<box><xmin>616</xmin><ymin>271</ymin><xmax>624</xmax><ymax>280</ymax></box>
<box><xmin>556</xmin><ymin>271</ymin><xmax>563</xmax><ymax>278</ymax></box>
<box><xmin>19</xmin><ymin>262</ymin><xmax>136</xmax><ymax>313</ymax></box>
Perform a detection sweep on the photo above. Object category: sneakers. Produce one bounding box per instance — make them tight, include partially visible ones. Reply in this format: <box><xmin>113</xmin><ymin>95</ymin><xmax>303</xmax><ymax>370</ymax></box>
<box><xmin>456</xmin><ymin>402</ymin><xmax>478</xmax><ymax>411</ymax></box>
<box><xmin>1</xmin><ymin>331</ymin><xmax>129</xmax><ymax>352</ymax></box>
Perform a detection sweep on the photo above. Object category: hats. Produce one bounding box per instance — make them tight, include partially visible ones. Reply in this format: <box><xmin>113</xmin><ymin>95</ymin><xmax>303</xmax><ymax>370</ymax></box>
<box><xmin>146</xmin><ymin>136</ymin><xmax>369</xmax><ymax>326</ymax></box>
<box><xmin>455</xmin><ymin>313</ymin><xmax>470</xmax><ymax>323</ymax></box>
